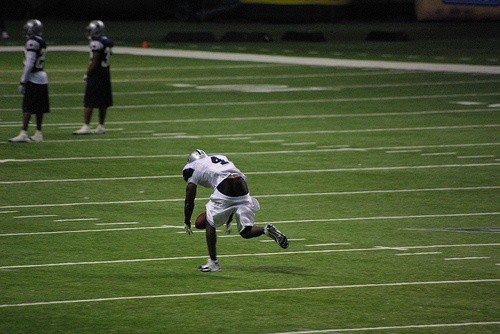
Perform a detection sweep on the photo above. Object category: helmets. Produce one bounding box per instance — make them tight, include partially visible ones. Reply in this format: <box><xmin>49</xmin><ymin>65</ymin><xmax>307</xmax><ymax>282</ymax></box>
<box><xmin>24</xmin><ymin>19</ymin><xmax>43</xmax><ymax>37</ymax></box>
<box><xmin>187</xmin><ymin>148</ymin><xmax>207</xmax><ymax>163</ymax></box>
<box><xmin>86</xmin><ymin>20</ymin><xmax>105</xmax><ymax>38</ymax></box>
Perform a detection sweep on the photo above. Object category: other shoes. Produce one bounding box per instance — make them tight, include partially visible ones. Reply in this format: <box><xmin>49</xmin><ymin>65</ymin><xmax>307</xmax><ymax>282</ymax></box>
<box><xmin>73</xmin><ymin>129</ymin><xmax>90</xmax><ymax>135</ymax></box>
<box><xmin>7</xmin><ymin>135</ymin><xmax>29</xmax><ymax>142</ymax></box>
<box><xmin>29</xmin><ymin>135</ymin><xmax>44</xmax><ymax>142</ymax></box>
<box><xmin>90</xmin><ymin>128</ymin><xmax>105</xmax><ymax>134</ymax></box>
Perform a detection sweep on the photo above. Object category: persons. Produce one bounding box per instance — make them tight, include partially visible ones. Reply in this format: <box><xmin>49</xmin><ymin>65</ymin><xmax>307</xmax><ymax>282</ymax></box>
<box><xmin>182</xmin><ymin>149</ymin><xmax>289</xmax><ymax>272</ymax></box>
<box><xmin>8</xmin><ymin>19</ymin><xmax>50</xmax><ymax>142</ymax></box>
<box><xmin>72</xmin><ymin>21</ymin><xmax>113</xmax><ymax>134</ymax></box>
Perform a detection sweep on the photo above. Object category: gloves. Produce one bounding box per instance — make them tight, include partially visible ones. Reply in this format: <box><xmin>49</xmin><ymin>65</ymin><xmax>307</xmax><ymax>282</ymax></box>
<box><xmin>225</xmin><ymin>219</ymin><xmax>234</xmax><ymax>235</ymax></box>
<box><xmin>183</xmin><ymin>222</ymin><xmax>194</xmax><ymax>236</ymax></box>
<box><xmin>17</xmin><ymin>85</ymin><xmax>26</xmax><ymax>94</ymax></box>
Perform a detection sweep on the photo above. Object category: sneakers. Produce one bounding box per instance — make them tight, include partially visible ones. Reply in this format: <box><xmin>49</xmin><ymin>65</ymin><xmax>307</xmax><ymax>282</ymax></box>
<box><xmin>264</xmin><ymin>223</ymin><xmax>290</xmax><ymax>249</ymax></box>
<box><xmin>199</xmin><ymin>259</ymin><xmax>222</xmax><ymax>273</ymax></box>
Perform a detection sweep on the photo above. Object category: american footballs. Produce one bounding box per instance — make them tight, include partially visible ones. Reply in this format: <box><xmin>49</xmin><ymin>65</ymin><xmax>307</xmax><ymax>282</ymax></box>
<box><xmin>195</xmin><ymin>212</ymin><xmax>207</xmax><ymax>230</ymax></box>
